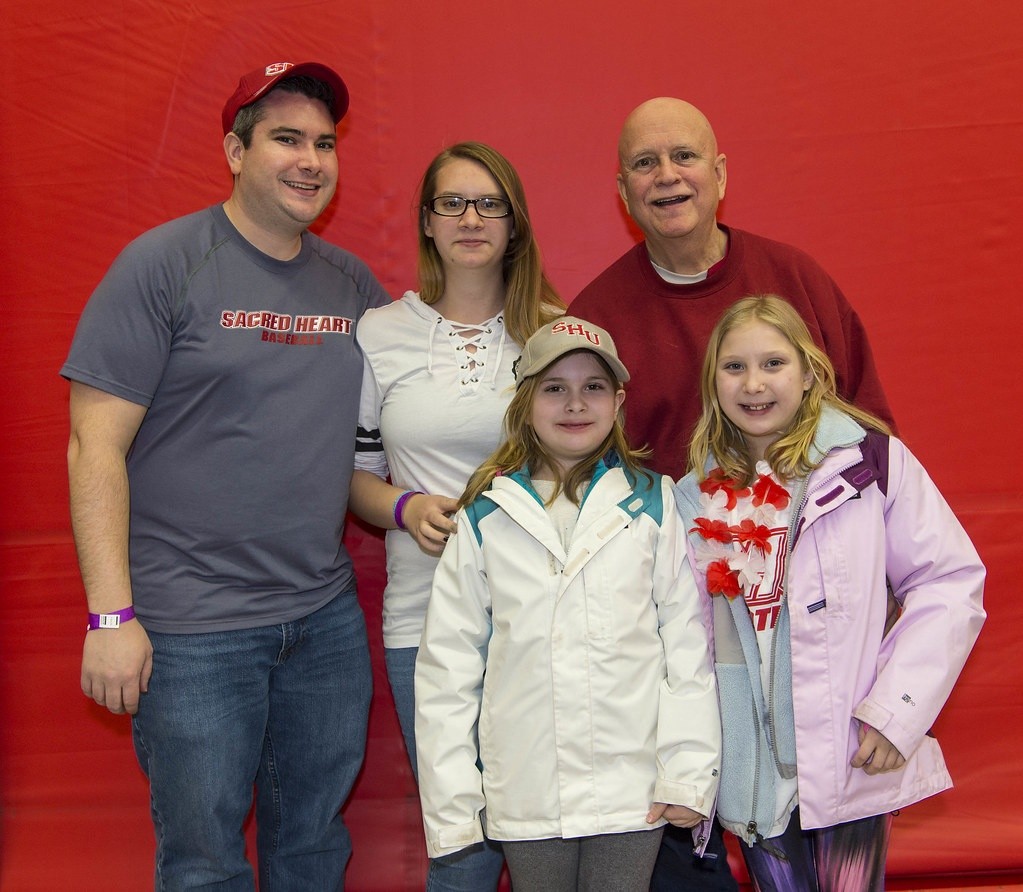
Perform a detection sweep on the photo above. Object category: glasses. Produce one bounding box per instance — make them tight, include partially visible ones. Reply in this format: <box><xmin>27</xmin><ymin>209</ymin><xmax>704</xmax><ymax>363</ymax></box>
<box><xmin>427</xmin><ymin>194</ymin><xmax>513</xmax><ymax>218</ymax></box>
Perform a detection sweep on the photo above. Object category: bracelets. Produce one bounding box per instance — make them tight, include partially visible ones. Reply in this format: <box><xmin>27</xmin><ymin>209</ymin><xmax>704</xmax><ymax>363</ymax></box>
<box><xmin>393</xmin><ymin>490</ymin><xmax>424</xmax><ymax>531</ymax></box>
<box><xmin>87</xmin><ymin>605</ymin><xmax>136</xmax><ymax>630</ymax></box>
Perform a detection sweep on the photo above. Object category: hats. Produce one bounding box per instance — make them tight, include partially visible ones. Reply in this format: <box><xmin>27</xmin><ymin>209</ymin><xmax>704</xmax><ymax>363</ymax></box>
<box><xmin>516</xmin><ymin>316</ymin><xmax>630</xmax><ymax>391</ymax></box>
<box><xmin>220</xmin><ymin>60</ymin><xmax>350</xmax><ymax>139</ymax></box>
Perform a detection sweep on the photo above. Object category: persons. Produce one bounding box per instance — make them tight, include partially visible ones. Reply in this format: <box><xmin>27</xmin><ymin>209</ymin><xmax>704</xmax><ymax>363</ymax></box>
<box><xmin>353</xmin><ymin>138</ymin><xmax>569</xmax><ymax>891</ymax></box>
<box><xmin>412</xmin><ymin>314</ymin><xmax>717</xmax><ymax>891</ymax></box>
<box><xmin>57</xmin><ymin>58</ymin><xmax>394</xmax><ymax>892</ymax></box>
<box><xmin>567</xmin><ymin>96</ymin><xmax>904</xmax><ymax>892</ymax></box>
<box><xmin>676</xmin><ymin>292</ymin><xmax>989</xmax><ymax>892</ymax></box>
